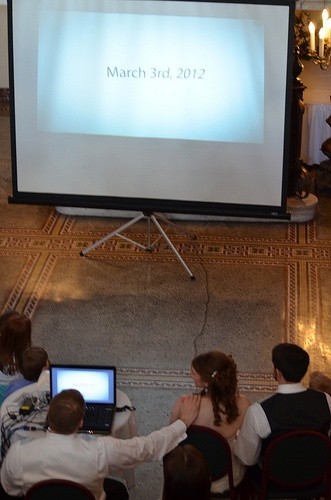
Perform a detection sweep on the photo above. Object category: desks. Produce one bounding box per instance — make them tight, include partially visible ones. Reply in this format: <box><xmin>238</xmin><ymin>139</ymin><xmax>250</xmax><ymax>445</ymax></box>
<box><xmin>0</xmin><ymin>379</ymin><xmax>136</xmax><ymax>488</ymax></box>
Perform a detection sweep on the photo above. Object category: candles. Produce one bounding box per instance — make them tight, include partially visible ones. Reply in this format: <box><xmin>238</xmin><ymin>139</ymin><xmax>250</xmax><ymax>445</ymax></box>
<box><xmin>308</xmin><ymin>9</ymin><xmax>331</xmax><ymax>56</ymax></box>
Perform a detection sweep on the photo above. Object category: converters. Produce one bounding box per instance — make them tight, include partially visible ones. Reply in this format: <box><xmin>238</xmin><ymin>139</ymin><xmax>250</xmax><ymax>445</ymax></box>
<box><xmin>19</xmin><ymin>397</ymin><xmax>37</xmax><ymax>415</ymax></box>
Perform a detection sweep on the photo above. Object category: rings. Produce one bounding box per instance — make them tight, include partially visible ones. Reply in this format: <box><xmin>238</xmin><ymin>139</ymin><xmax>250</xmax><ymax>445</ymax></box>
<box><xmin>194</xmin><ymin>397</ymin><xmax>198</xmax><ymax>400</ymax></box>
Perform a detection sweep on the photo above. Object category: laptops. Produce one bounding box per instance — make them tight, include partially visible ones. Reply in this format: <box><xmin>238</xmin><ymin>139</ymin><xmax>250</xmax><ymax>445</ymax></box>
<box><xmin>44</xmin><ymin>364</ymin><xmax>117</xmax><ymax>434</ymax></box>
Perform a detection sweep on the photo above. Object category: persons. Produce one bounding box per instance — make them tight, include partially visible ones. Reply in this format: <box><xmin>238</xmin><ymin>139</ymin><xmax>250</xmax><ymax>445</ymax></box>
<box><xmin>0</xmin><ymin>312</ymin><xmax>49</xmax><ymax>407</ymax></box>
<box><xmin>308</xmin><ymin>373</ymin><xmax>331</xmax><ymax>395</ymax></box>
<box><xmin>169</xmin><ymin>351</ymin><xmax>251</xmax><ymax>494</ymax></box>
<box><xmin>0</xmin><ymin>390</ymin><xmax>200</xmax><ymax>500</ymax></box>
<box><xmin>231</xmin><ymin>343</ymin><xmax>331</xmax><ymax>492</ymax></box>
